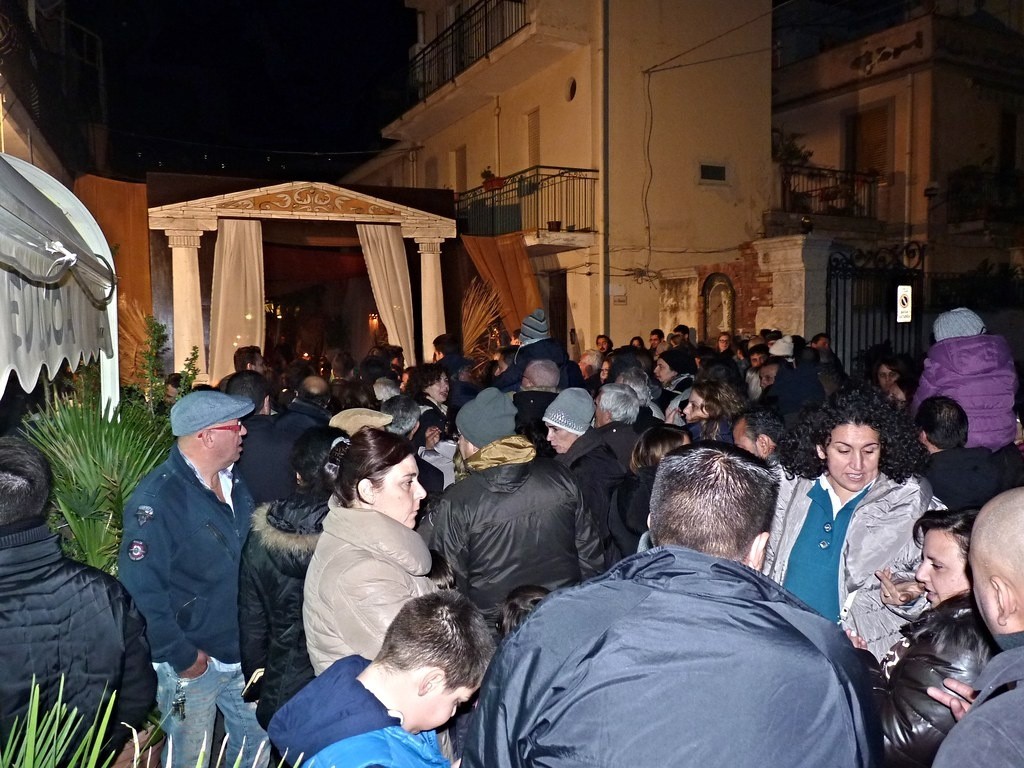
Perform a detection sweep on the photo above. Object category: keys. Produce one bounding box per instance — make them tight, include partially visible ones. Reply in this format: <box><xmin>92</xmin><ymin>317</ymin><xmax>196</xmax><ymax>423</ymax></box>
<box><xmin>171</xmin><ymin>680</ymin><xmax>187</xmax><ymax>721</ymax></box>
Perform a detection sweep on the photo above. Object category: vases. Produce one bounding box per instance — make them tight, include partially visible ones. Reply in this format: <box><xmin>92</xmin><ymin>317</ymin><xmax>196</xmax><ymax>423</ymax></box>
<box><xmin>483</xmin><ymin>177</ymin><xmax>505</xmax><ymax>191</ymax></box>
<box><xmin>548</xmin><ymin>221</ymin><xmax>562</xmax><ymax>232</ymax></box>
<box><xmin>829</xmin><ymin>207</ymin><xmax>850</xmax><ymax>216</ymax></box>
<box><xmin>566</xmin><ymin>226</ymin><xmax>576</xmax><ymax>231</ymax></box>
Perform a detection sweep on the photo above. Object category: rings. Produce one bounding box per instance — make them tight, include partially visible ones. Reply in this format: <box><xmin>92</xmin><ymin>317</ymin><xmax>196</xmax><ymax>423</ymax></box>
<box><xmin>883</xmin><ymin>594</ymin><xmax>891</xmax><ymax>598</ymax></box>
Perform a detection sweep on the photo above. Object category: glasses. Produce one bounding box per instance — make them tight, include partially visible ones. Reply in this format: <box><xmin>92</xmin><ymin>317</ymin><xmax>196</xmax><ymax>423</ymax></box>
<box><xmin>198</xmin><ymin>421</ymin><xmax>241</xmax><ymax>438</ymax></box>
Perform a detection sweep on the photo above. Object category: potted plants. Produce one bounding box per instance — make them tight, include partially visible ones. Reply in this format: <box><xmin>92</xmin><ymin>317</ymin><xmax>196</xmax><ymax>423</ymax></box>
<box><xmin>516</xmin><ymin>174</ymin><xmax>538</xmax><ymax>197</ymax></box>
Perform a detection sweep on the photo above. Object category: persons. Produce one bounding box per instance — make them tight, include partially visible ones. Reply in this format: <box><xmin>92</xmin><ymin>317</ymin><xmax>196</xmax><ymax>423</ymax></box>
<box><xmin>0</xmin><ymin>302</ymin><xmax>1024</xmax><ymax>768</ymax></box>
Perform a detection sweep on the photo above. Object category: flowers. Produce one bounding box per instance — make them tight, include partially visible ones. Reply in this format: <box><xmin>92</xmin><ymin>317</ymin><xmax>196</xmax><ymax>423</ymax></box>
<box><xmin>816</xmin><ymin>185</ymin><xmax>860</xmax><ymax>204</ymax></box>
<box><xmin>479</xmin><ymin>166</ymin><xmax>500</xmax><ymax>180</ymax></box>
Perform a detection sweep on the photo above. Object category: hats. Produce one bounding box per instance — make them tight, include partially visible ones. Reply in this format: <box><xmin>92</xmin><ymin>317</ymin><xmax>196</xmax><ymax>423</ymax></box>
<box><xmin>456</xmin><ymin>387</ymin><xmax>518</xmax><ymax>449</ymax></box>
<box><xmin>769</xmin><ymin>335</ymin><xmax>793</xmax><ymax>356</ymax></box>
<box><xmin>171</xmin><ymin>391</ymin><xmax>255</xmax><ymax>436</ymax></box>
<box><xmin>542</xmin><ymin>388</ymin><xmax>595</xmax><ymax>436</ymax></box>
<box><xmin>519</xmin><ymin>309</ymin><xmax>548</xmax><ymax>342</ymax></box>
<box><xmin>329</xmin><ymin>408</ymin><xmax>392</xmax><ymax>437</ymax></box>
<box><xmin>933</xmin><ymin>307</ymin><xmax>987</xmax><ymax>342</ymax></box>
<box><xmin>658</xmin><ymin>350</ymin><xmax>689</xmax><ymax>374</ymax></box>
